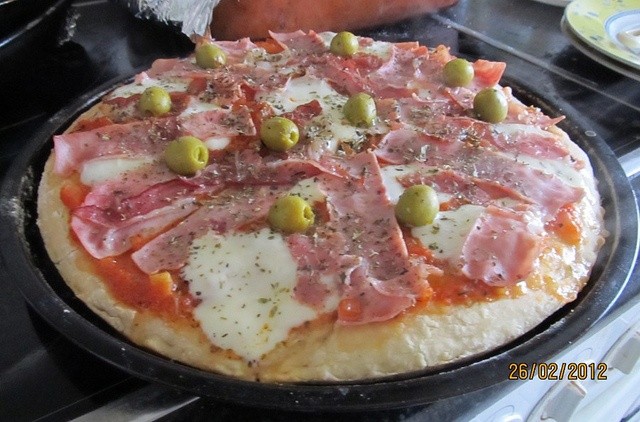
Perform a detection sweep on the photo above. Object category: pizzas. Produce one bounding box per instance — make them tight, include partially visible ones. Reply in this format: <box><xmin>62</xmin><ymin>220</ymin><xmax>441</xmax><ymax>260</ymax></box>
<box><xmin>36</xmin><ymin>28</ymin><xmax>606</xmax><ymax>385</ymax></box>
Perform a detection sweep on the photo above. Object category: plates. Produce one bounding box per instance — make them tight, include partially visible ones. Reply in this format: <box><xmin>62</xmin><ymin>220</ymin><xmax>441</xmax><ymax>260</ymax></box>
<box><xmin>563</xmin><ymin>0</ymin><xmax>640</xmax><ymax>71</ymax></box>
<box><xmin>559</xmin><ymin>13</ymin><xmax>640</xmax><ymax>82</ymax></box>
<box><xmin>1</xmin><ymin>55</ymin><xmax>636</xmax><ymax>409</ymax></box>
